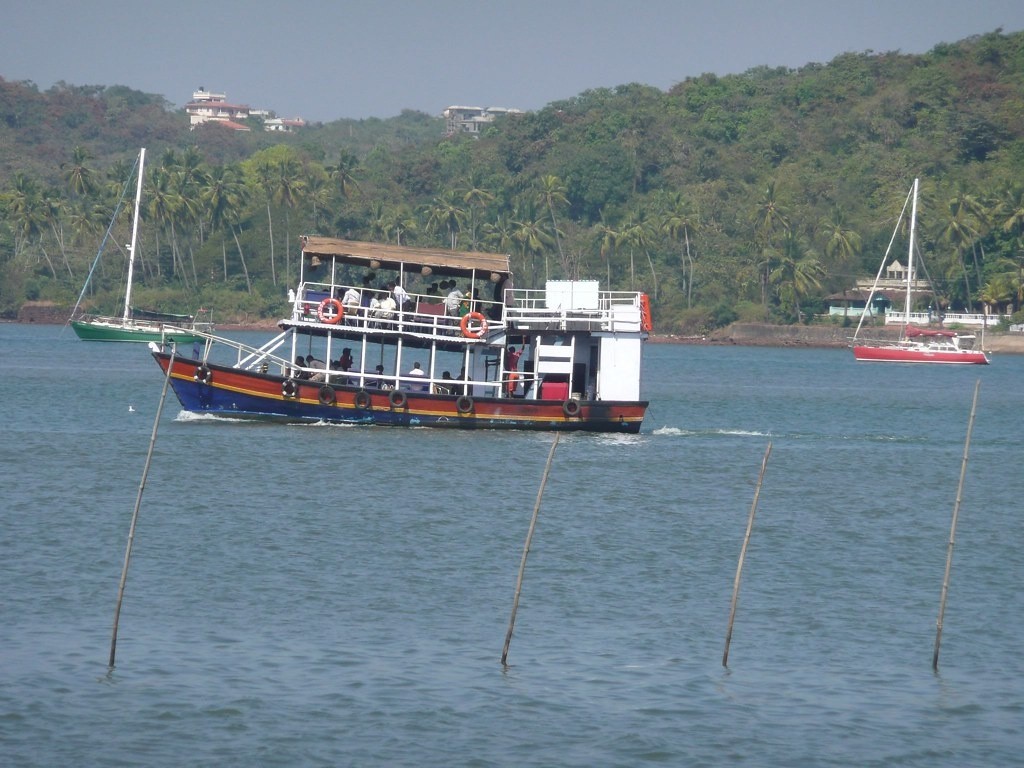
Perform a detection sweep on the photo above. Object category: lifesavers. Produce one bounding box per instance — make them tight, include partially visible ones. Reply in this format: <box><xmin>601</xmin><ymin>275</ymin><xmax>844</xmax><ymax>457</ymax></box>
<box><xmin>354</xmin><ymin>392</ymin><xmax>372</xmax><ymax>409</ymax></box>
<box><xmin>389</xmin><ymin>389</ymin><xmax>407</xmax><ymax>408</ymax></box>
<box><xmin>640</xmin><ymin>293</ymin><xmax>652</xmax><ymax>332</ymax></box>
<box><xmin>562</xmin><ymin>399</ymin><xmax>581</xmax><ymax>417</ymax></box>
<box><xmin>456</xmin><ymin>396</ymin><xmax>473</xmax><ymax>413</ymax></box>
<box><xmin>317</xmin><ymin>298</ymin><xmax>344</xmax><ymax>324</ymax></box>
<box><xmin>460</xmin><ymin>313</ymin><xmax>488</xmax><ymax>338</ymax></box>
<box><xmin>281</xmin><ymin>380</ymin><xmax>298</xmax><ymax>398</ymax></box>
<box><xmin>318</xmin><ymin>385</ymin><xmax>336</xmax><ymax>405</ymax></box>
<box><xmin>193</xmin><ymin>366</ymin><xmax>212</xmax><ymax>385</ymax></box>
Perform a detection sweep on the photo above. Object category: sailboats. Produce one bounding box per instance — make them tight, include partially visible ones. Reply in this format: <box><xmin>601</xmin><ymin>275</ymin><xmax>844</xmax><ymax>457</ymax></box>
<box><xmin>847</xmin><ymin>177</ymin><xmax>991</xmax><ymax>365</ymax></box>
<box><xmin>64</xmin><ymin>147</ymin><xmax>216</xmax><ymax>344</ymax></box>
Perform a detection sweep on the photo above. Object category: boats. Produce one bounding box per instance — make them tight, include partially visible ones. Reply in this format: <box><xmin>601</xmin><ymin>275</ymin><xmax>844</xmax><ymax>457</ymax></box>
<box><xmin>148</xmin><ymin>235</ymin><xmax>654</xmax><ymax>434</ymax></box>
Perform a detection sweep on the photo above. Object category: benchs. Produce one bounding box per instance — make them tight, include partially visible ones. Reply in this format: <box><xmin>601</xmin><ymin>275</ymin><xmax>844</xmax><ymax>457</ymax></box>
<box><xmin>414</xmin><ymin>303</ymin><xmax>446</xmax><ymax>335</ymax></box>
<box><xmin>398</xmin><ymin>374</ymin><xmax>430</xmax><ymax>393</ymax></box>
<box><xmin>347</xmin><ymin>368</ymin><xmax>379</xmax><ymax>389</ymax></box>
<box><xmin>305</xmin><ymin>291</ymin><xmax>369</xmax><ymax>326</ymax></box>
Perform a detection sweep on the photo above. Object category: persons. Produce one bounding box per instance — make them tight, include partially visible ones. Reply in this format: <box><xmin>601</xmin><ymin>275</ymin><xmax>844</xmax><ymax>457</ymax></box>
<box><xmin>409</xmin><ymin>362</ymin><xmax>424</xmax><ymax>391</ymax></box>
<box><xmin>295</xmin><ymin>348</ymin><xmax>384</xmax><ymax>389</ymax></box>
<box><xmin>323</xmin><ymin>273</ymin><xmax>481</xmax><ymax>337</ymax></box>
<box><xmin>507</xmin><ymin>334</ymin><xmax>526</xmax><ymax>398</ymax></box>
<box><xmin>441</xmin><ymin>367</ymin><xmax>473</xmax><ymax>397</ymax></box>
